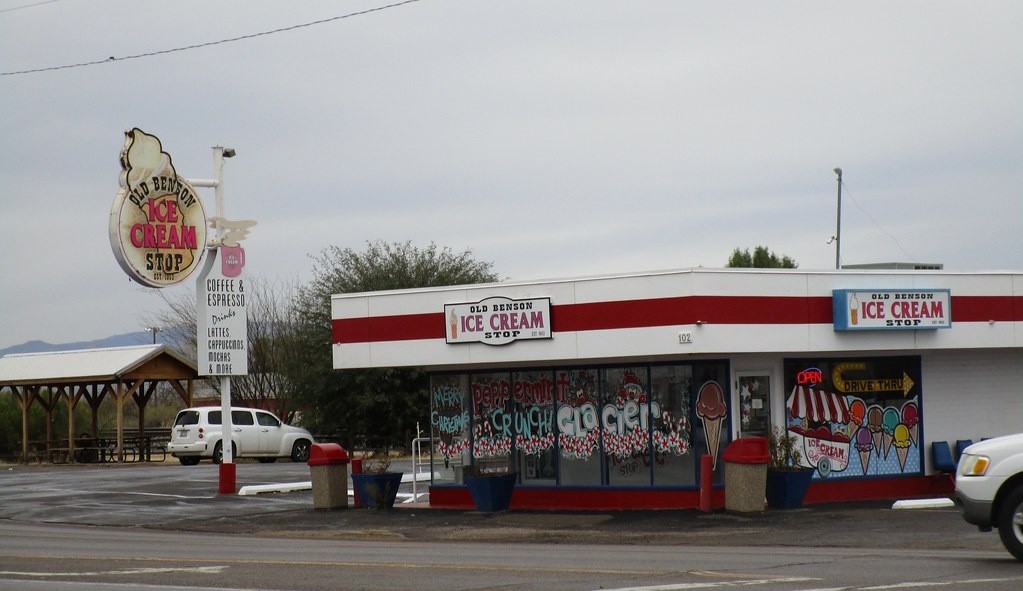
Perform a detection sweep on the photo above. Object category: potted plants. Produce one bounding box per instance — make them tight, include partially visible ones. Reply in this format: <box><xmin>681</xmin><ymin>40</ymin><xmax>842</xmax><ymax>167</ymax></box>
<box><xmin>462</xmin><ymin>453</ymin><xmax>517</xmax><ymax>512</ymax></box>
<box><xmin>765</xmin><ymin>428</ymin><xmax>815</xmax><ymax>508</ymax></box>
<box><xmin>351</xmin><ymin>446</ymin><xmax>403</xmax><ymax>510</ymax></box>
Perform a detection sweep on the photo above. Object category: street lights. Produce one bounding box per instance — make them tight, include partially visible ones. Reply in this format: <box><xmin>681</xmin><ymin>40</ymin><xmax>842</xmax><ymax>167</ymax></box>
<box><xmin>211</xmin><ymin>144</ymin><xmax>238</xmax><ymax>495</ymax></box>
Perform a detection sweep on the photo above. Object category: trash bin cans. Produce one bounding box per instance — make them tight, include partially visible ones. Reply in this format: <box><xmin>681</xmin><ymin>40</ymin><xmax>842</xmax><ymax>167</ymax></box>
<box><xmin>307</xmin><ymin>443</ymin><xmax>350</xmax><ymax>509</ymax></box>
<box><xmin>721</xmin><ymin>437</ymin><xmax>772</xmax><ymax>513</ymax></box>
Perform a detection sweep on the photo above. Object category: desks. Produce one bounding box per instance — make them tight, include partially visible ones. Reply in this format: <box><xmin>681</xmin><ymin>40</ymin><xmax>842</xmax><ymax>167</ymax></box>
<box><xmin>62</xmin><ymin>436</ymin><xmax>156</xmax><ymax>464</ymax></box>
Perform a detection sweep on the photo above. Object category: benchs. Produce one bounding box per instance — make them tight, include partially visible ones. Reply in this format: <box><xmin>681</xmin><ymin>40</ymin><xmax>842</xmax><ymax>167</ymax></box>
<box><xmin>931</xmin><ymin>437</ymin><xmax>991</xmax><ymax>497</ymax></box>
<box><xmin>49</xmin><ymin>445</ymin><xmax>168</xmax><ymax>465</ymax></box>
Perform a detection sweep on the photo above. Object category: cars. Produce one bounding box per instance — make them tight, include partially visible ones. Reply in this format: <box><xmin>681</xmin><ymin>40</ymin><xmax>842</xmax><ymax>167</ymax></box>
<box><xmin>948</xmin><ymin>432</ymin><xmax>1023</xmax><ymax>563</ymax></box>
<box><xmin>169</xmin><ymin>407</ymin><xmax>315</xmax><ymax>465</ymax></box>
